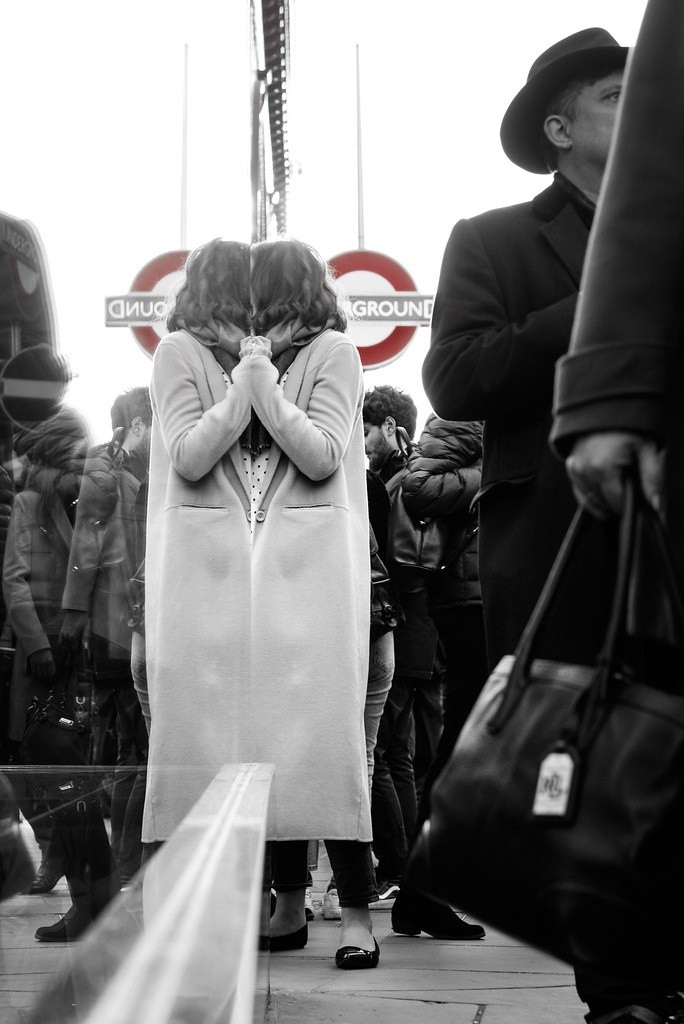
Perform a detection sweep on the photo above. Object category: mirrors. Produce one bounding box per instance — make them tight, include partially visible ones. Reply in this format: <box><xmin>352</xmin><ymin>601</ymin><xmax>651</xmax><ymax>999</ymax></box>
<box><xmin>0</xmin><ymin>0</ymin><xmax>264</xmax><ymax>1023</ymax></box>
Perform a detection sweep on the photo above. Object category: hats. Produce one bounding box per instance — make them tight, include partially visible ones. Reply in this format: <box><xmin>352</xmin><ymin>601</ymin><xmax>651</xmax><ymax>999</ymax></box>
<box><xmin>500</xmin><ymin>27</ymin><xmax>630</xmax><ymax>174</ymax></box>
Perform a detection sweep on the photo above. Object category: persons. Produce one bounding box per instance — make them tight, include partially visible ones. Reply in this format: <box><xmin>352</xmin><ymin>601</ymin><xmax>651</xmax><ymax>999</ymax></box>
<box><xmin>0</xmin><ymin>240</ymin><xmax>250</xmax><ymax>943</ymax></box>
<box><xmin>266</xmin><ymin>387</ymin><xmax>488</xmax><ymax>937</ymax></box>
<box><xmin>421</xmin><ymin>1</ymin><xmax>684</xmax><ymax>1024</ymax></box>
<box><xmin>249</xmin><ymin>237</ymin><xmax>381</xmax><ymax>968</ymax></box>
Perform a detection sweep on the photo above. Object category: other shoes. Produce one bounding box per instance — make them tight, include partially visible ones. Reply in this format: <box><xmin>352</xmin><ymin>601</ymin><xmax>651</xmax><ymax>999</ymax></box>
<box><xmin>21</xmin><ymin>853</ymin><xmax>63</xmax><ymax>892</ymax></box>
<box><xmin>336</xmin><ymin>936</ymin><xmax>380</xmax><ymax>970</ymax></box>
<box><xmin>368</xmin><ymin>880</ymin><xmax>399</xmax><ymax>910</ymax></box>
<box><xmin>35</xmin><ymin>891</ymin><xmax>122</xmax><ymax>942</ymax></box>
<box><xmin>392</xmin><ymin>880</ymin><xmax>486</xmax><ymax>940</ymax></box>
<box><xmin>319</xmin><ymin>889</ymin><xmax>341</xmax><ymax>919</ymax></box>
<box><xmin>305</xmin><ymin>889</ymin><xmax>315</xmax><ymax>920</ymax></box>
<box><xmin>269</xmin><ymin>924</ymin><xmax>308</xmax><ymax>952</ymax></box>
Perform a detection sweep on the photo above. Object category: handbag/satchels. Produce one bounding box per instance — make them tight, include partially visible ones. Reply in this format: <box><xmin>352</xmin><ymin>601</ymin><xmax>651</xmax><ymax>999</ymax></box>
<box><xmin>20</xmin><ymin>649</ymin><xmax>109</xmax><ymax>801</ymax></box>
<box><xmin>408</xmin><ymin>475</ymin><xmax>684</xmax><ymax>978</ymax></box>
<box><xmin>385</xmin><ymin>426</ymin><xmax>448</xmax><ymax>569</ymax></box>
